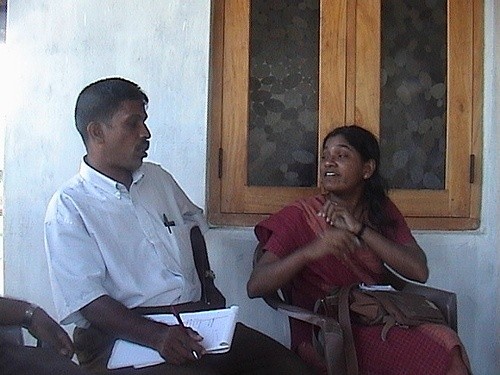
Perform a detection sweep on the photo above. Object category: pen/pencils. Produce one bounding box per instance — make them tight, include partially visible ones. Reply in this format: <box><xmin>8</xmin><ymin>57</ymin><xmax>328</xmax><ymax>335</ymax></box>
<box><xmin>163</xmin><ymin>213</ymin><xmax>172</xmax><ymax>233</ymax></box>
<box><xmin>171</xmin><ymin>306</ymin><xmax>199</xmax><ymax>361</ymax></box>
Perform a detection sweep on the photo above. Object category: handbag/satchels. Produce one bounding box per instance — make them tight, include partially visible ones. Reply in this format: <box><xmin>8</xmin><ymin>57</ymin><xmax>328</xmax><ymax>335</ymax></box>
<box><xmin>313</xmin><ymin>281</ymin><xmax>446</xmax><ymax>375</ymax></box>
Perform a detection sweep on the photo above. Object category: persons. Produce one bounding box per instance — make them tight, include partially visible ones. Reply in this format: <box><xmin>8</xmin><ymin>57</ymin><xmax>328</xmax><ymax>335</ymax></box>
<box><xmin>0</xmin><ymin>297</ymin><xmax>82</xmax><ymax>375</ymax></box>
<box><xmin>43</xmin><ymin>77</ymin><xmax>317</xmax><ymax>375</ymax></box>
<box><xmin>247</xmin><ymin>125</ymin><xmax>472</xmax><ymax>375</ymax></box>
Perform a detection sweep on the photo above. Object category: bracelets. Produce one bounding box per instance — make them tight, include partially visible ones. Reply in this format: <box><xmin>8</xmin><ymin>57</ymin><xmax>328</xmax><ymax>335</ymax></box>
<box><xmin>355</xmin><ymin>223</ymin><xmax>367</xmax><ymax>239</ymax></box>
<box><xmin>205</xmin><ymin>269</ymin><xmax>215</xmax><ymax>279</ymax></box>
<box><xmin>22</xmin><ymin>303</ymin><xmax>39</xmax><ymax>329</ymax></box>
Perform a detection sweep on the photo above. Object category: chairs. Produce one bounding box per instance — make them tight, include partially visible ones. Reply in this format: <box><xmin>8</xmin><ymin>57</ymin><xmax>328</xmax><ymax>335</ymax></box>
<box><xmin>253</xmin><ymin>244</ymin><xmax>458</xmax><ymax>375</ymax></box>
